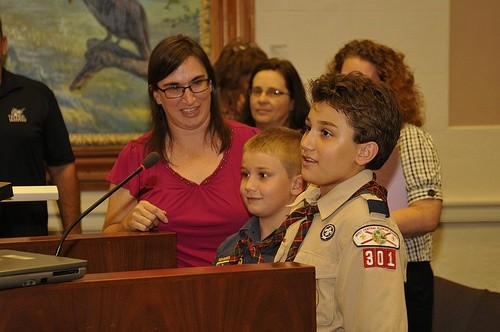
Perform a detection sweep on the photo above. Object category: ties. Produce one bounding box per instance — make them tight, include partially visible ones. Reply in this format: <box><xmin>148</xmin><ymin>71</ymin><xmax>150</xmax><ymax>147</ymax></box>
<box><xmin>254</xmin><ymin>172</ymin><xmax>388</xmax><ymax>262</ymax></box>
<box><xmin>228</xmin><ymin>236</ymin><xmax>277</xmax><ymax>265</ymax></box>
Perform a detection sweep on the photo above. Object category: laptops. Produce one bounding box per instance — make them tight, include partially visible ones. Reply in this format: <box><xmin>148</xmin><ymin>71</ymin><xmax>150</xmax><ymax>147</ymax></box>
<box><xmin>0</xmin><ymin>249</ymin><xmax>87</xmax><ymax>290</ymax></box>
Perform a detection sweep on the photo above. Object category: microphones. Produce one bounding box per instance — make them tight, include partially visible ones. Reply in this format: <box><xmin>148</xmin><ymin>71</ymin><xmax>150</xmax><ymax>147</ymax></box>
<box><xmin>55</xmin><ymin>152</ymin><xmax>159</xmax><ymax>256</ymax></box>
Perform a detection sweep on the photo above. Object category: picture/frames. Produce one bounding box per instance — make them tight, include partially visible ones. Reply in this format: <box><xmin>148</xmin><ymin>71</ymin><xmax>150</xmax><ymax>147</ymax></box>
<box><xmin>0</xmin><ymin>0</ymin><xmax>255</xmax><ymax>192</ymax></box>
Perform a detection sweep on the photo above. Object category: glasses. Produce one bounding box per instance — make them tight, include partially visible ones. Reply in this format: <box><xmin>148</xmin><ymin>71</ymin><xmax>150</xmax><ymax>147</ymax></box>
<box><xmin>154</xmin><ymin>77</ymin><xmax>212</xmax><ymax>99</ymax></box>
<box><xmin>249</xmin><ymin>87</ymin><xmax>290</xmax><ymax>97</ymax></box>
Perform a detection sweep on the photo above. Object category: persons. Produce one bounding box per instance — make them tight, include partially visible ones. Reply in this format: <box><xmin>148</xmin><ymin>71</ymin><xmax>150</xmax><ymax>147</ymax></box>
<box><xmin>0</xmin><ymin>17</ymin><xmax>82</xmax><ymax>240</ymax></box>
<box><xmin>273</xmin><ymin>71</ymin><xmax>409</xmax><ymax>332</ymax></box>
<box><xmin>213</xmin><ymin>126</ymin><xmax>319</xmax><ymax>268</ymax></box>
<box><xmin>237</xmin><ymin>57</ymin><xmax>311</xmax><ymax>136</ymax></box>
<box><xmin>210</xmin><ymin>37</ymin><xmax>268</xmax><ymax>123</ymax></box>
<box><xmin>325</xmin><ymin>39</ymin><xmax>444</xmax><ymax>332</ymax></box>
<box><xmin>102</xmin><ymin>34</ymin><xmax>262</xmax><ymax>268</ymax></box>
